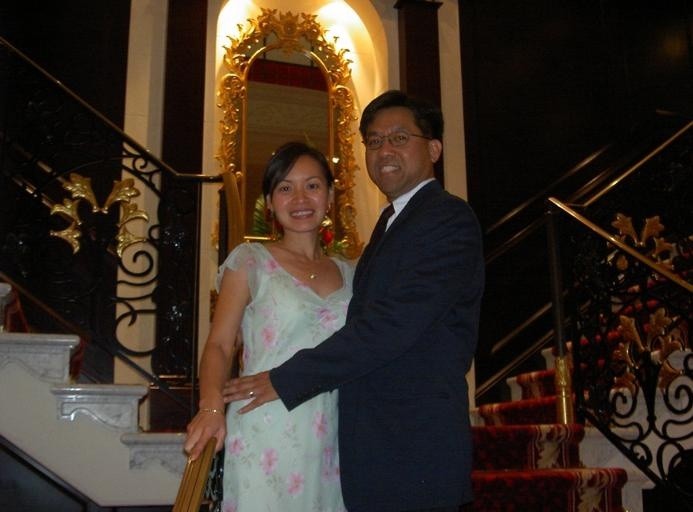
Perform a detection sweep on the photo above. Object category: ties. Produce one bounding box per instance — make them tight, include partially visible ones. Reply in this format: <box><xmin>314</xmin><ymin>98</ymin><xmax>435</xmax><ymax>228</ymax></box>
<box><xmin>371</xmin><ymin>204</ymin><xmax>395</xmax><ymax>251</ymax></box>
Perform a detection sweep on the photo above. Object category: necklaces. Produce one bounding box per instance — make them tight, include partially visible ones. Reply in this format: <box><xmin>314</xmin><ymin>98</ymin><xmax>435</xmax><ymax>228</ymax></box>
<box><xmin>274</xmin><ymin>239</ymin><xmax>324</xmax><ymax>280</ymax></box>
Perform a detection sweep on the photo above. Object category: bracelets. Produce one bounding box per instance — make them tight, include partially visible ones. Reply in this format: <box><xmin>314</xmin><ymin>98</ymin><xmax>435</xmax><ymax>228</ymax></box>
<box><xmin>198</xmin><ymin>408</ymin><xmax>224</xmax><ymax>415</ymax></box>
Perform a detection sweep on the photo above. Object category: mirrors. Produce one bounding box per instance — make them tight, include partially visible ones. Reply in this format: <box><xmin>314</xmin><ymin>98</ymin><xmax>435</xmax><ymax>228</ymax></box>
<box><xmin>215</xmin><ymin>6</ymin><xmax>367</xmax><ymax>261</ymax></box>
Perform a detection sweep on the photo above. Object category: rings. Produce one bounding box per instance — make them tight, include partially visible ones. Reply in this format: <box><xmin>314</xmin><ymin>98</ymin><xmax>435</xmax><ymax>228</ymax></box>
<box><xmin>248</xmin><ymin>388</ymin><xmax>254</xmax><ymax>398</ymax></box>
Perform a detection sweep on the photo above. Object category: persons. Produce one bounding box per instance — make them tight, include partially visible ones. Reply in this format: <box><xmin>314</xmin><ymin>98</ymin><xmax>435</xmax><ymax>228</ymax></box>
<box><xmin>220</xmin><ymin>90</ymin><xmax>485</xmax><ymax>511</ymax></box>
<box><xmin>180</xmin><ymin>140</ymin><xmax>359</xmax><ymax>512</ymax></box>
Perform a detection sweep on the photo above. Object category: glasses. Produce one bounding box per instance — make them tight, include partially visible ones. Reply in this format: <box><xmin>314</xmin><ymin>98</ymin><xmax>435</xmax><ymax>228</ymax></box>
<box><xmin>362</xmin><ymin>131</ymin><xmax>432</xmax><ymax>151</ymax></box>
